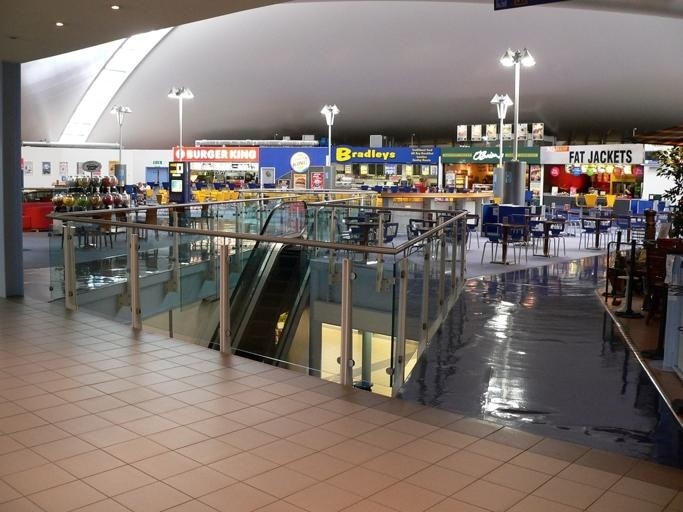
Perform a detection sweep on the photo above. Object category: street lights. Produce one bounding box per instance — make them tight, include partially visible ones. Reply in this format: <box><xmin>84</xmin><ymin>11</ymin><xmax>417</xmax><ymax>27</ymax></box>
<box><xmin>319</xmin><ymin>101</ymin><xmax>341</xmax><ymax>190</ymax></box>
<box><xmin>165</xmin><ymin>84</ymin><xmax>196</xmax><ymax>160</ymax></box>
<box><xmin>109</xmin><ymin>99</ymin><xmax>134</xmax><ymax>197</ymax></box>
<box><xmin>487</xmin><ymin>89</ymin><xmax>514</xmax><ymax>169</ymax></box>
<box><xmin>496</xmin><ymin>44</ymin><xmax>537</xmax><ymax>162</ymax></box>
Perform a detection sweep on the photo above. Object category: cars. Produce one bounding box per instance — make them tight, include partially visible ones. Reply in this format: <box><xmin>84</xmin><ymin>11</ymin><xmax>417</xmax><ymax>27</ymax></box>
<box><xmin>21</xmin><ymin>185</ymin><xmax>68</xmax><ymax>231</ymax></box>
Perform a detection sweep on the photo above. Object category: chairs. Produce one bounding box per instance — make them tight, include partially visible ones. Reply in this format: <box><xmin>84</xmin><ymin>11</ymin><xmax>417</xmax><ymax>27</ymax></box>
<box><xmin>144</xmin><ymin>249</ymin><xmax>157</xmax><ymax>272</ymax></box>
<box><xmin>92</xmin><ymin>204</ymin><xmax>209</xmax><ymax>249</ymax></box>
<box><xmin>99</xmin><ymin>258</ymin><xmax>112</xmax><ymax>276</ymax></box>
<box><xmin>123</xmin><ymin>181</ymin><xmax>275</xmax><ymax>204</ymax></box>
